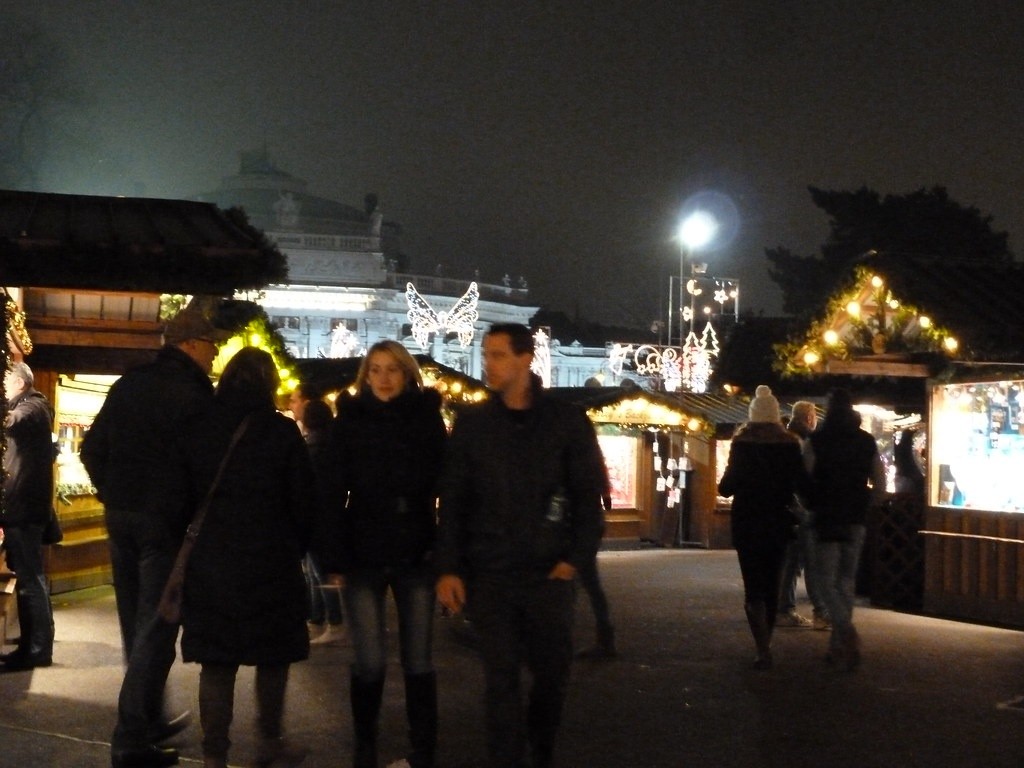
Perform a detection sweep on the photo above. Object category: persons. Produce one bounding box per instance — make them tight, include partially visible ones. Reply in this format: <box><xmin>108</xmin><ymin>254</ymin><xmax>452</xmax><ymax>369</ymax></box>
<box><xmin>715</xmin><ymin>383</ymin><xmax>921</xmax><ymax>668</ymax></box>
<box><xmin>77</xmin><ymin>314</ymin><xmax>624</xmax><ymax>768</ymax></box>
<box><xmin>0</xmin><ymin>362</ymin><xmax>54</xmax><ymax>673</ymax></box>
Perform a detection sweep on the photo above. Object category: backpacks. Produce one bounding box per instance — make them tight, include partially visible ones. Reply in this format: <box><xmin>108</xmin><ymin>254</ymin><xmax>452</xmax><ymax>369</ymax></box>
<box><xmin>156</xmin><ymin>536</ymin><xmax>193</xmax><ymax>623</ymax></box>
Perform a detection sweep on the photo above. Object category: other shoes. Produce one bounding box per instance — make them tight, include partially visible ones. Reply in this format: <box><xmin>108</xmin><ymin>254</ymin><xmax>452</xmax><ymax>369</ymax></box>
<box><xmin>253</xmin><ymin>736</ymin><xmax>307</xmax><ymax>768</ymax></box>
<box><xmin>204</xmin><ymin>754</ymin><xmax>227</xmax><ymax>768</ymax></box>
<box><xmin>574</xmin><ymin>638</ymin><xmax>617</xmax><ymax>662</ymax></box>
<box><xmin>0</xmin><ymin>633</ymin><xmax>53</xmax><ymax>671</ymax></box>
<box><xmin>774</xmin><ymin>610</ymin><xmax>833</xmax><ymax>631</ymax></box>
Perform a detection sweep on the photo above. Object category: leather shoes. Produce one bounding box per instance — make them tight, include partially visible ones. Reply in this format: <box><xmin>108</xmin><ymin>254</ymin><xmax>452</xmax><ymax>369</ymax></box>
<box><xmin>110</xmin><ymin>709</ymin><xmax>190</xmax><ymax>768</ymax></box>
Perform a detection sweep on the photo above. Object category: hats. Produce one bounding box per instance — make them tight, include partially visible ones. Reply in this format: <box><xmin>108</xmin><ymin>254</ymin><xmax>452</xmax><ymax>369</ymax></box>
<box><xmin>749</xmin><ymin>385</ymin><xmax>780</xmax><ymax>423</ymax></box>
<box><xmin>161</xmin><ymin>312</ymin><xmax>229</xmax><ymax>346</ymax></box>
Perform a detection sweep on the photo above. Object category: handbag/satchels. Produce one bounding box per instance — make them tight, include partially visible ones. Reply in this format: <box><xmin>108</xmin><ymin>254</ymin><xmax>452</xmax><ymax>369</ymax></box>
<box><xmin>41</xmin><ymin>508</ymin><xmax>63</xmax><ymax>545</ymax></box>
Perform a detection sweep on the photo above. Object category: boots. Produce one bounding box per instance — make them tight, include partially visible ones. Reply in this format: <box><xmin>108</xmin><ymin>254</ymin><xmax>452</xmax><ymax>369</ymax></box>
<box><xmin>743</xmin><ymin>602</ymin><xmax>777</xmax><ymax>668</ymax></box>
